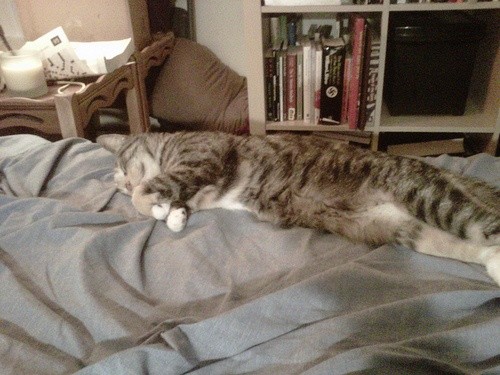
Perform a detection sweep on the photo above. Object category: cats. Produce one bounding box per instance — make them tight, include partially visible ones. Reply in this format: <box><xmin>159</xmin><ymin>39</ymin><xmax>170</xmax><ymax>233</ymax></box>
<box><xmin>96</xmin><ymin>130</ymin><xmax>500</xmax><ymax>286</ymax></box>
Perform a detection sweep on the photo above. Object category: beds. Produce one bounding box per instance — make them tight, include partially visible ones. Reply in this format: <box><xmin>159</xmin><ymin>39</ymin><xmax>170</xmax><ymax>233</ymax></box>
<box><xmin>0</xmin><ymin>134</ymin><xmax>500</xmax><ymax>373</ymax></box>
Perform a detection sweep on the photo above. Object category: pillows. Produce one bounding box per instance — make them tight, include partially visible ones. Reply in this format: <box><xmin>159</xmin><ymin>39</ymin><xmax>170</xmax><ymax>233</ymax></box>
<box><xmin>145</xmin><ymin>33</ymin><xmax>250</xmax><ymax>136</ymax></box>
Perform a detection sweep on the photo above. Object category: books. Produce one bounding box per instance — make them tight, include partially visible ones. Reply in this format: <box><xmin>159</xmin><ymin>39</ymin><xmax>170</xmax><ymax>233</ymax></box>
<box><xmin>264</xmin><ymin>15</ymin><xmax>382</xmax><ymax>130</ymax></box>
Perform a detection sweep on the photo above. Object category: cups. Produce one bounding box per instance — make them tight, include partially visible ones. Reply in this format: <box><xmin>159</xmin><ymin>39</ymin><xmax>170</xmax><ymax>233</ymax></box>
<box><xmin>1</xmin><ymin>49</ymin><xmax>49</xmax><ymax>98</ymax></box>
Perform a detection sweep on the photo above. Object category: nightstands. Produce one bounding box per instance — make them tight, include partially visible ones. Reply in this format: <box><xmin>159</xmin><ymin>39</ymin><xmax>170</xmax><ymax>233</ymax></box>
<box><xmin>0</xmin><ymin>39</ymin><xmax>153</xmax><ymax>139</ymax></box>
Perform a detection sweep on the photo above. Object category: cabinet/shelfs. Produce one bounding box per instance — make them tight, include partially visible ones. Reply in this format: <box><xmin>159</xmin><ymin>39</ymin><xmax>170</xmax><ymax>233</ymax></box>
<box><xmin>245</xmin><ymin>0</ymin><xmax>500</xmax><ymax>159</ymax></box>
